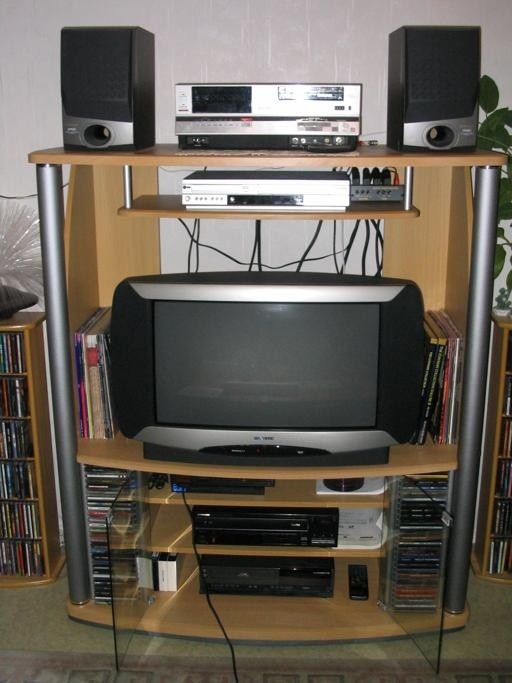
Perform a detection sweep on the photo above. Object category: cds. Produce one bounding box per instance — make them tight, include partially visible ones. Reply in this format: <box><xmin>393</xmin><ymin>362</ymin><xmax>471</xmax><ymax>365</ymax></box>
<box><xmin>324</xmin><ymin>479</ymin><xmax>364</xmax><ymax>491</ymax></box>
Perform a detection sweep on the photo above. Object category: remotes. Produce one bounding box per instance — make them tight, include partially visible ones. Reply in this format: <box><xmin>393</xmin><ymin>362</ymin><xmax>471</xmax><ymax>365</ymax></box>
<box><xmin>348</xmin><ymin>565</ymin><xmax>369</xmax><ymax>600</ymax></box>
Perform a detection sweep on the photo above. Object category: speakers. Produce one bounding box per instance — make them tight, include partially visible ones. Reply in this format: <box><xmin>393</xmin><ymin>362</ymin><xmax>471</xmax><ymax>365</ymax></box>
<box><xmin>60</xmin><ymin>25</ymin><xmax>155</xmax><ymax>151</ymax></box>
<box><xmin>389</xmin><ymin>26</ymin><xmax>480</xmax><ymax>153</ymax></box>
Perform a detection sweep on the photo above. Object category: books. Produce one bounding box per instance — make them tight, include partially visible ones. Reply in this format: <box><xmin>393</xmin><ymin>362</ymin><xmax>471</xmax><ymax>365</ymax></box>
<box><xmin>488</xmin><ymin>331</ymin><xmax>512</xmax><ymax>576</ymax></box>
<box><xmin>74</xmin><ymin>303</ymin><xmax>117</xmax><ymax>442</ymax></box>
<box><xmin>410</xmin><ymin>306</ymin><xmax>461</xmax><ymax>447</ymax></box>
<box><xmin>1</xmin><ymin>331</ymin><xmax>49</xmax><ymax>576</ymax></box>
<box><xmin>386</xmin><ymin>471</ymin><xmax>449</xmax><ymax>613</ymax></box>
<box><xmin>83</xmin><ymin>462</ymin><xmax>145</xmax><ymax>606</ymax></box>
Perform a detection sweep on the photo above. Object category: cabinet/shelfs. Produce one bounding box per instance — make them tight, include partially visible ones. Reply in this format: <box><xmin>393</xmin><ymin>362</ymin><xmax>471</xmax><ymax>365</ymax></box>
<box><xmin>471</xmin><ymin>313</ymin><xmax>512</xmax><ymax>584</ymax></box>
<box><xmin>28</xmin><ymin>143</ymin><xmax>507</xmax><ymax>644</ymax></box>
<box><xmin>0</xmin><ymin>310</ymin><xmax>67</xmax><ymax>585</ymax></box>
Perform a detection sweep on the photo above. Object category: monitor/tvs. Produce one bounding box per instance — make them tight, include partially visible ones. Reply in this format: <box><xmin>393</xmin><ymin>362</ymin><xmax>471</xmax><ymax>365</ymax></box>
<box><xmin>108</xmin><ymin>271</ymin><xmax>425</xmax><ymax>467</ymax></box>
